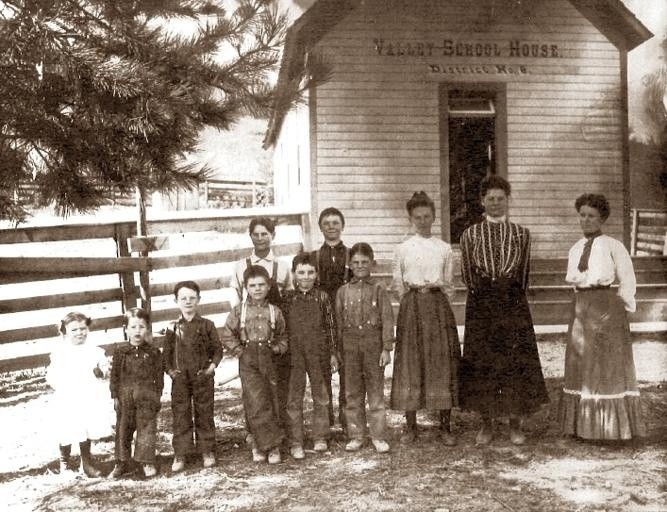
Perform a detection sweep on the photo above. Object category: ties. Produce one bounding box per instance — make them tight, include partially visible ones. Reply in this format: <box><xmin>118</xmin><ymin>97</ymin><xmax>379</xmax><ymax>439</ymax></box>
<box><xmin>577</xmin><ymin>236</ymin><xmax>595</xmax><ymax>273</ymax></box>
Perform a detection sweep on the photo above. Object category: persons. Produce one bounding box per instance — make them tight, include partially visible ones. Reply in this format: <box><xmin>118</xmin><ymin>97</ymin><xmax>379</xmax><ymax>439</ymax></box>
<box><xmin>558</xmin><ymin>194</ymin><xmax>647</xmax><ymax>442</ymax></box>
<box><xmin>109</xmin><ymin>307</ymin><xmax>164</xmax><ymax>478</ymax></box>
<box><xmin>228</xmin><ymin>217</ymin><xmax>293</xmax><ymax>443</ymax></box>
<box><xmin>458</xmin><ymin>176</ymin><xmax>552</xmax><ymax>445</ymax></box>
<box><xmin>310</xmin><ymin>208</ymin><xmax>352</xmax><ymax>438</ymax></box>
<box><xmin>222</xmin><ymin>265</ymin><xmax>288</xmax><ymax>465</ymax></box>
<box><xmin>390</xmin><ymin>192</ymin><xmax>460</xmax><ymax>447</ymax></box>
<box><xmin>162</xmin><ymin>282</ymin><xmax>223</xmax><ymax>471</ymax></box>
<box><xmin>336</xmin><ymin>243</ymin><xmax>394</xmax><ymax>453</ymax></box>
<box><xmin>45</xmin><ymin>313</ymin><xmax>109</xmax><ymax>477</ymax></box>
<box><xmin>282</xmin><ymin>253</ymin><xmax>338</xmax><ymax>460</ymax></box>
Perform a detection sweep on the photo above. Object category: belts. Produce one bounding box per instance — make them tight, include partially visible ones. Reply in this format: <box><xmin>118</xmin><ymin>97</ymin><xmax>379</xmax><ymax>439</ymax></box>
<box><xmin>409</xmin><ymin>286</ymin><xmax>441</xmax><ymax>293</ymax></box>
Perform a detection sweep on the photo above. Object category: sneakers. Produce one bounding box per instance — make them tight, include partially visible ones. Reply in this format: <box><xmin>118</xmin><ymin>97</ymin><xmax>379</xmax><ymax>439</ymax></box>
<box><xmin>400</xmin><ymin>424</ymin><xmax>419</xmax><ymax>444</ymax></box>
<box><xmin>437</xmin><ymin>425</ymin><xmax>457</xmax><ymax>446</ymax></box>
<box><xmin>202</xmin><ymin>450</ymin><xmax>216</xmax><ymax>466</ymax></box>
<box><xmin>250</xmin><ymin>446</ymin><xmax>266</xmax><ymax>462</ymax></box>
<box><xmin>344</xmin><ymin>436</ymin><xmax>367</xmax><ymax>452</ymax></box>
<box><xmin>475</xmin><ymin>423</ymin><xmax>494</xmax><ymax>443</ymax></box>
<box><xmin>312</xmin><ymin>439</ymin><xmax>329</xmax><ymax>452</ymax></box>
<box><xmin>509</xmin><ymin>425</ymin><xmax>526</xmax><ymax>445</ymax></box>
<box><xmin>142</xmin><ymin>463</ymin><xmax>158</xmax><ymax>477</ymax></box>
<box><xmin>171</xmin><ymin>456</ymin><xmax>186</xmax><ymax>472</ymax></box>
<box><xmin>371</xmin><ymin>438</ymin><xmax>390</xmax><ymax>453</ymax></box>
<box><xmin>267</xmin><ymin>445</ymin><xmax>282</xmax><ymax>464</ymax></box>
<box><xmin>290</xmin><ymin>444</ymin><xmax>305</xmax><ymax>459</ymax></box>
<box><xmin>107</xmin><ymin>462</ymin><xmax>131</xmax><ymax>480</ymax></box>
